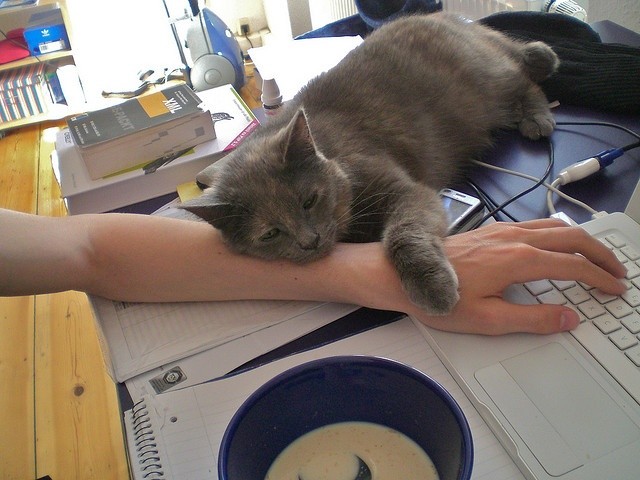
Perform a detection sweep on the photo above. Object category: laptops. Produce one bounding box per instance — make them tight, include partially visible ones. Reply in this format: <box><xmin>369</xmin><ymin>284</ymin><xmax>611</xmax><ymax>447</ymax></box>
<box><xmin>407</xmin><ymin>180</ymin><xmax>639</xmax><ymax>480</ymax></box>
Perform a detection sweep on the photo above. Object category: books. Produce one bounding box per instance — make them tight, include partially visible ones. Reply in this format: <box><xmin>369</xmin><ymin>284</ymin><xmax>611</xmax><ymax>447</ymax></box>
<box><xmin>50</xmin><ymin>81</ymin><xmax>260</xmax><ymax>217</ymax></box>
<box><xmin>246</xmin><ymin>34</ymin><xmax>364</xmax><ymax>105</ymax></box>
<box><xmin>0</xmin><ymin>56</ymin><xmax>87</xmax><ymax>122</ymax></box>
<box><xmin>65</xmin><ymin>84</ymin><xmax>217</xmax><ymax>183</ymax></box>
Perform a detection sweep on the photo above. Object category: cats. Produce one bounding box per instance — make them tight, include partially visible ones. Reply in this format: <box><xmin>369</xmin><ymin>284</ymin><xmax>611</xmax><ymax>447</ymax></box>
<box><xmin>174</xmin><ymin>11</ymin><xmax>561</xmax><ymax>317</ymax></box>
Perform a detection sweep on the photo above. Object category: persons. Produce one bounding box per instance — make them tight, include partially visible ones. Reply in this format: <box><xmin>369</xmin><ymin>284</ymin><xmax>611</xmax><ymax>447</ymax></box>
<box><xmin>0</xmin><ymin>207</ymin><xmax>628</xmax><ymax>338</ymax></box>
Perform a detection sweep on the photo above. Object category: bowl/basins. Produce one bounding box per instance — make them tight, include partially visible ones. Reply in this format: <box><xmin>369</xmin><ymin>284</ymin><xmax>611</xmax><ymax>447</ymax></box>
<box><xmin>217</xmin><ymin>354</ymin><xmax>473</xmax><ymax>480</ymax></box>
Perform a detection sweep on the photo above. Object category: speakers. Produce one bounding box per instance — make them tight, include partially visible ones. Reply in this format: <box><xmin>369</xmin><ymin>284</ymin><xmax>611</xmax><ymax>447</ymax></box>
<box><xmin>187</xmin><ymin>8</ymin><xmax>244</xmax><ymax>91</ymax></box>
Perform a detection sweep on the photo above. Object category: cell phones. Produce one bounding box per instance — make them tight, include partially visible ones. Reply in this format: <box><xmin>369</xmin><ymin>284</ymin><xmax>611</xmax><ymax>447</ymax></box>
<box><xmin>440</xmin><ymin>189</ymin><xmax>484</xmax><ymax>234</ymax></box>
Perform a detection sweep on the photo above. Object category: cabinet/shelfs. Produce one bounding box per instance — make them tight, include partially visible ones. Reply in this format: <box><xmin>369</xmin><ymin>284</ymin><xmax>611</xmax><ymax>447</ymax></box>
<box><xmin>0</xmin><ymin>0</ymin><xmax>86</xmax><ymax>130</ymax></box>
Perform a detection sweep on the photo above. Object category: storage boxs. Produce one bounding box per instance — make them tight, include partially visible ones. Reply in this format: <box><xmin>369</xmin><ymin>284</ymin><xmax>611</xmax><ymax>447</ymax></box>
<box><xmin>23</xmin><ymin>9</ymin><xmax>70</xmax><ymax>56</ymax></box>
<box><xmin>0</xmin><ymin>62</ymin><xmax>53</xmax><ymax>122</ymax></box>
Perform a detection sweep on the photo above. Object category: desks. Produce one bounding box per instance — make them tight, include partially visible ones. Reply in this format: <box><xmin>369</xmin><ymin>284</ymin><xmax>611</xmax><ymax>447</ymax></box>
<box><xmin>88</xmin><ymin>18</ymin><xmax>640</xmax><ymax>480</ymax></box>
